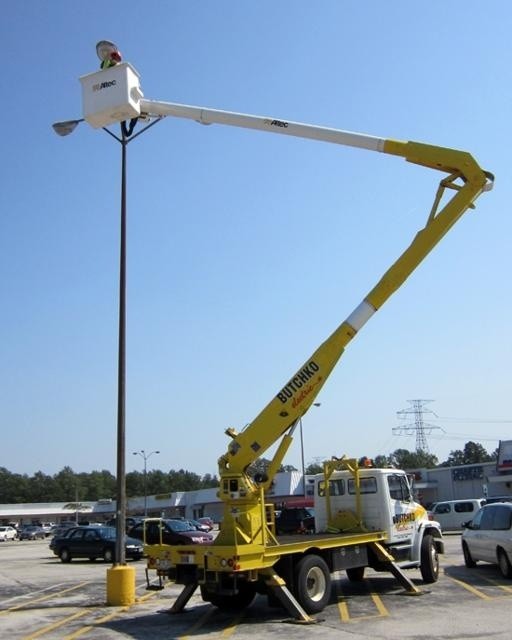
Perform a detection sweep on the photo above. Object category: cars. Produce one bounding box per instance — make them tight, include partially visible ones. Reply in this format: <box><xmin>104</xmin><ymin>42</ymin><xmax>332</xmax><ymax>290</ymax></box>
<box><xmin>129</xmin><ymin>517</ymin><xmax>213</xmax><ymax>545</ymax></box>
<box><xmin>48</xmin><ymin>525</ymin><xmax>149</xmax><ymax>561</ymax></box>
<box><xmin>106</xmin><ymin>517</ymin><xmax>142</xmax><ymax>532</ymax></box>
<box><xmin>460</xmin><ymin>502</ymin><xmax>512</xmax><ymax>582</ymax></box>
<box><xmin>0</xmin><ymin>522</ymin><xmax>78</xmax><ymax>541</ymax></box>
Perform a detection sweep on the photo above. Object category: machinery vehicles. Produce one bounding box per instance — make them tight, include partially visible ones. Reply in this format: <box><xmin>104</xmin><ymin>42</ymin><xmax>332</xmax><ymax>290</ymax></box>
<box><xmin>55</xmin><ymin>39</ymin><xmax>496</xmax><ymax>626</ymax></box>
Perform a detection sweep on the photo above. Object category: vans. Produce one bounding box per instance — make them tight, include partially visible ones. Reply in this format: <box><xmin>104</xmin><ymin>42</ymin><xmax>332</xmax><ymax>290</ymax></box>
<box><xmin>423</xmin><ymin>498</ymin><xmax>486</xmax><ymax>532</ymax></box>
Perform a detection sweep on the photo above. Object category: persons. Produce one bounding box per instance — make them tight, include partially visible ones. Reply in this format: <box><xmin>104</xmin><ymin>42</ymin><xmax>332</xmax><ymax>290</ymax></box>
<box><xmin>106</xmin><ymin>52</ymin><xmax>121</xmax><ymax>68</ymax></box>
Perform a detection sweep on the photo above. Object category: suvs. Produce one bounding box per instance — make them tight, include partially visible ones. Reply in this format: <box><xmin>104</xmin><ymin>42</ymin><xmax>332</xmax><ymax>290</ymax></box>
<box><xmin>266</xmin><ymin>507</ymin><xmax>314</xmax><ymax>535</ymax></box>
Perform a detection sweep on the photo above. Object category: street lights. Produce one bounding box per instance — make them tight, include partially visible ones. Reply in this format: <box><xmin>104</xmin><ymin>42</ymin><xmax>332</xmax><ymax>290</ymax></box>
<box><xmin>51</xmin><ymin>113</ymin><xmax>164</xmax><ymax>564</ymax></box>
<box><xmin>300</xmin><ymin>403</ymin><xmax>323</xmax><ymax>475</ymax></box>
<box><xmin>132</xmin><ymin>451</ymin><xmax>161</xmax><ymax>516</ymax></box>
